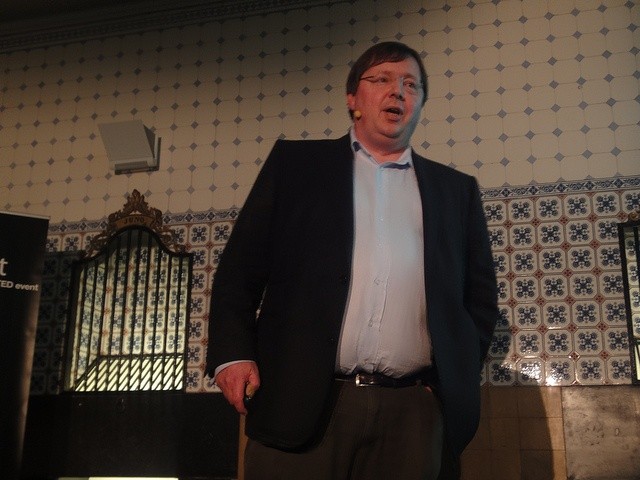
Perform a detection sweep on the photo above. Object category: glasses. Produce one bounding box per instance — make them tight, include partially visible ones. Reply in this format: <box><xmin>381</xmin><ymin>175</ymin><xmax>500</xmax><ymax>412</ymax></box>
<box><xmin>359</xmin><ymin>74</ymin><xmax>424</xmax><ymax>94</ymax></box>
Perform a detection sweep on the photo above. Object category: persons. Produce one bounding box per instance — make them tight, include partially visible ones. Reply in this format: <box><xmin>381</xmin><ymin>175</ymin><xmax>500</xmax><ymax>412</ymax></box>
<box><xmin>206</xmin><ymin>42</ymin><xmax>500</xmax><ymax>480</ymax></box>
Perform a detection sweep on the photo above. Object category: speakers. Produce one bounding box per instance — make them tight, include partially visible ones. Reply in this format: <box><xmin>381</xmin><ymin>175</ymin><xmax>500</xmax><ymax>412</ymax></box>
<box><xmin>99</xmin><ymin>120</ymin><xmax>159</xmax><ymax>172</ymax></box>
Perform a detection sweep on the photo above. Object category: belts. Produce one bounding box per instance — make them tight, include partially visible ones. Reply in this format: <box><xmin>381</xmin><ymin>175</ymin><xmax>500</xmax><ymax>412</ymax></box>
<box><xmin>336</xmin><ymin>371</ymin><xmax>418</xmax><ymax>389</ymax></box>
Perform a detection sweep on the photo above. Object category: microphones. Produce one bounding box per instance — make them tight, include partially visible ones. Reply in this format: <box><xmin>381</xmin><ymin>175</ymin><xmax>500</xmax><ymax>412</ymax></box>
<box><xmin>354</xmin><ymin>111</ymin><xmax>362</xmax><ymax>118</ymax></box>
